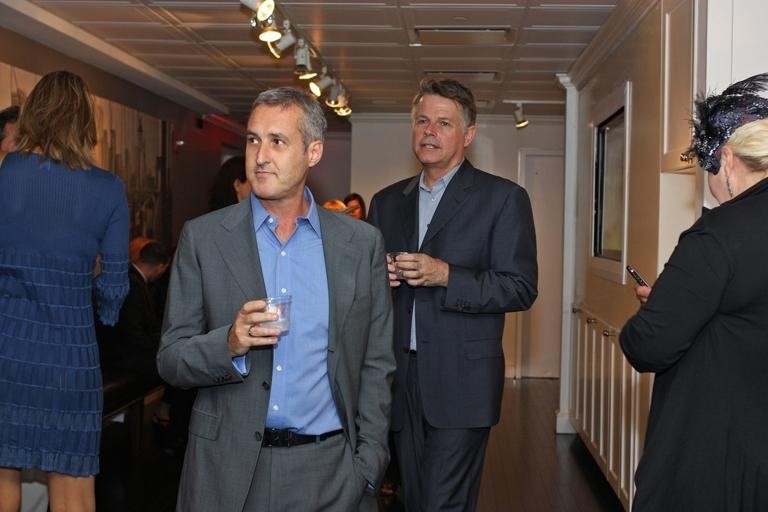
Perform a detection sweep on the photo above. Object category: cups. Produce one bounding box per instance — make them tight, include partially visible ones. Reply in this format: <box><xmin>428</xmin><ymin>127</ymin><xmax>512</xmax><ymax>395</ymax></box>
<box><xmin>260</xmin><ymin>294</ymin><xmax>291</xmax><ymax>332</ymax></box>
<box><xmin>389</xmin><ymin>252</ymin><xmax>407</xmax><ymax>280</ymax></box>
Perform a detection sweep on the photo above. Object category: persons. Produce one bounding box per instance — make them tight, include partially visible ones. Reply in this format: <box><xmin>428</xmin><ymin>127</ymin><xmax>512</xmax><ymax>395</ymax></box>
<box><xmin>157</xmin><ymin>88</ymin><xmax>397</xmax><ymax>512</ymax></box>
<box><xmin>617</xmin><ymin>71</ymin><xmax>767</xmax><ymax>512</ymax></box>
<box><xmin>0</xmin><ymin>104</ymin><xmax>25</xmax><ymax>158</ymax></box>
<box><xmin>368</xmin><ymin>75</ymin><xmax>541</xmax><ymax>510</ymax></box>
<box><xmin>89</xmin><ymin>153</ymin><xmax>366</xmax><ymax>512</ymax></box>
<box><xmin>0</xmin><ymin>69</ymin><xmax>130</xmax><ymax>511</ymax></box>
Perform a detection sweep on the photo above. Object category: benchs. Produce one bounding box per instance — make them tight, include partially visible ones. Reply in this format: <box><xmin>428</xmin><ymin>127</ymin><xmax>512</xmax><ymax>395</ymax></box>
<box><xmin>103</xmin><ymin>359</ymin><xmax>162</xmax><ymax>458</ymax></box>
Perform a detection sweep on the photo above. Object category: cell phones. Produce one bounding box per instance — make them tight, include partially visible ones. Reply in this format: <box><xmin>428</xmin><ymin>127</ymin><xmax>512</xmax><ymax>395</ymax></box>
<box><xmin>627</xmin><ymin>266</ymin><xmax>649</xmax><ymax>287</ymax></box>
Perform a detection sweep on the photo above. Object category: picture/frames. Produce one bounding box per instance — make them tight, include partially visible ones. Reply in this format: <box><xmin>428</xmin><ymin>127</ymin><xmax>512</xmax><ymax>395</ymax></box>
<box><xmin>586</xmin><ymin>80</ymin><xmax>632</xmax><ymax>285</ymax></box>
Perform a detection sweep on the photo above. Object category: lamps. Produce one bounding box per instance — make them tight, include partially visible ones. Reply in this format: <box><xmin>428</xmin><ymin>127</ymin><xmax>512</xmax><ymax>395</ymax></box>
<box><xmin>201</xmin><ymin>113</ymin><xmax>245</xmax><ymax>137</ymax></box>
<box><xmin>514</xmin><ymin>104</ymin><xmax>529</xmax><ymax>128</ymax></box>
<box><xmin>240</xmin><ymin>0</ymin><xmax>353</xmax><ymax>116</ymax></box>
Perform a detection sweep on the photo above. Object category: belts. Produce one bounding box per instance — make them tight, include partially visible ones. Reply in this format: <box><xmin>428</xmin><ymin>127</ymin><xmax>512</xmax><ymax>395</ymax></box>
<box><xmin>262</xmin><ymin>428</ymin><xmax>343</xmax><ymax>447</ymax></box>
